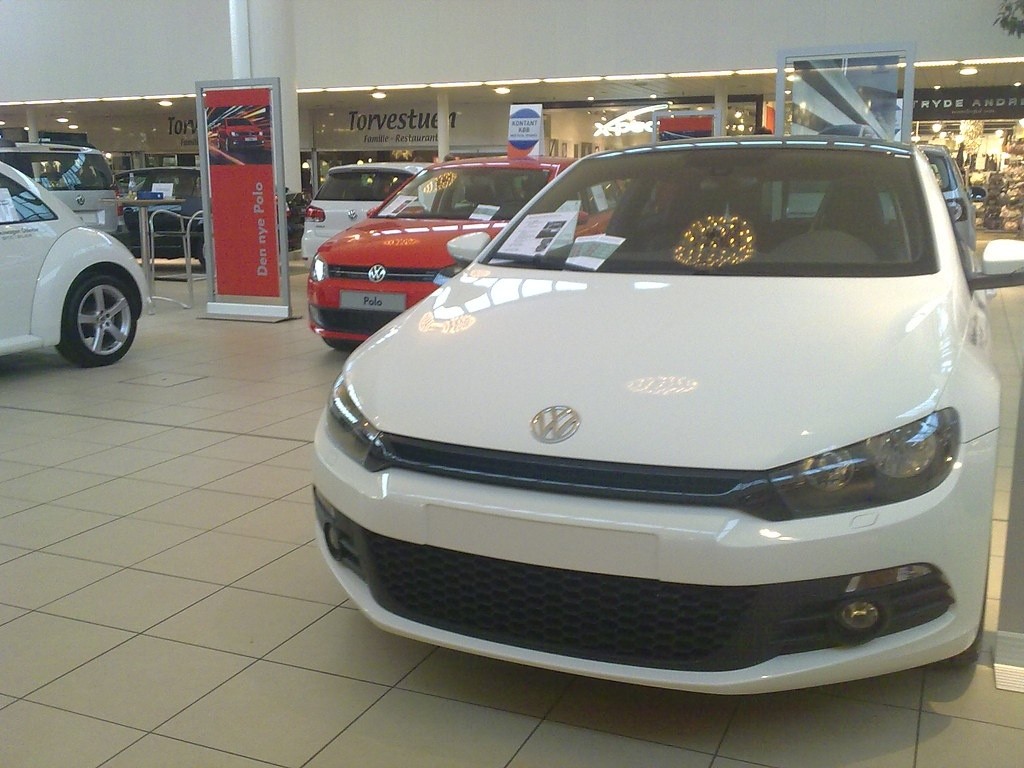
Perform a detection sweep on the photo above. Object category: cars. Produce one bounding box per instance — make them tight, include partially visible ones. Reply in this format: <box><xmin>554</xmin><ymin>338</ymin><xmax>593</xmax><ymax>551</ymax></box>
<box><xmin>108</xmin><ymin>167</ymin><xmax>304</xmax><ymax>270</ymax></box>
<box><xmin>818</xmin><ymin>124</ymin><xmax>876</xmax><ymax>138</ymax></box>
<box><xmin>313</xmin><ymin>134</ymin><xmax>1024</xmax><ymax>694</ymax></box>
<box><xmin>308</xmin><ymin>156</ymin><xmax>619</xmax><ymax>350</ymax></box>
<box><xmin>917</xmin><ymin>143</ymin><xmax>987</xmax><ymax>252</ymax></box>
<box><xmin>216</xmin><ymin>118</ymin><xmax>265</xmax><ymax>153</ymax></box>
<box><xmin>0</xmin><ymin>161</ymin><xmax>149</xmax><ymax>368</ymax></box>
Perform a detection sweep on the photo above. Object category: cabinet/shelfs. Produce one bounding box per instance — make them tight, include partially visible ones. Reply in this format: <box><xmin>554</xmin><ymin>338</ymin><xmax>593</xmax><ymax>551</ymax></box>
<box><xmin>959</xmin><ymin>156</ymin><xmax>1024</xmax><ymax>233</ymax></box>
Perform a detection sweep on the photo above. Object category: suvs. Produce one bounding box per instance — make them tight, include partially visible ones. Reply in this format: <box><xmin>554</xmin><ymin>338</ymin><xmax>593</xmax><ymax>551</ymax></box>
<box><xmin>301</xmin><ymin>163</ymin><xmax>439</xmax><ymax>270</ymax></box>
<box><xmin>0</xmin><ymin>138</ymin><xmax>131</xmax><ymax>250</ymax></box>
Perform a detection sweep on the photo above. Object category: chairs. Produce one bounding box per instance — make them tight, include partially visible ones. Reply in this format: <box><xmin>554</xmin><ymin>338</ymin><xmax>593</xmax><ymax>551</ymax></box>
<box><xmin>628</xmin><ymin>185</ymin><xmax>725</xmax><ymax>242</ymax></box>
<box><xmin>453</xmin><ymin>184</ymin><xmax>494</xmax><ymax>219</ymax></box>
<box><xmin>810</xmin><ymin>176</ymin><xmax>887</xmax><ymax>244</ymax></box>
